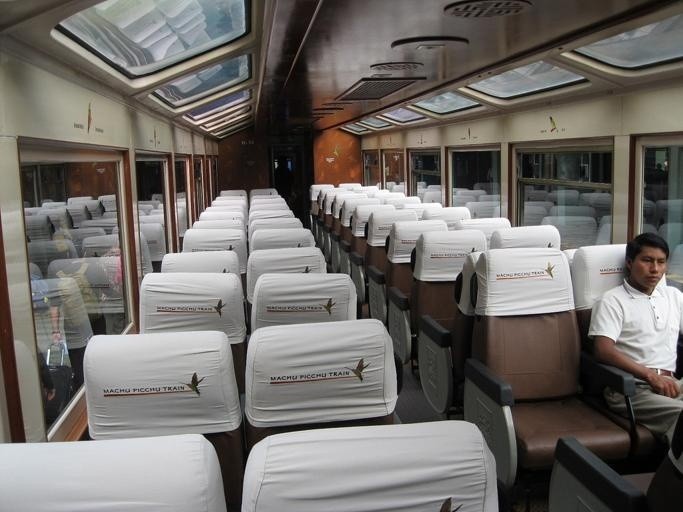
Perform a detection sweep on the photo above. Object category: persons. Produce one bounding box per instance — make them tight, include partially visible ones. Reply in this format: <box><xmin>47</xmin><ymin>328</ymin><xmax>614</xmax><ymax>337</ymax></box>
<box><xmin>275</xmin><ymin>158</ymin><xmax>297</xmax><ymax>211</ymax></box>
<box><xmin>588</xmin><ymin>233</ymin><xmax>683</xmax><ymax>443</ymax></box>
<box><xmin>29</xmin><ymin>215</ymin><xmax>122</xmax><ymax>421</ymax></box>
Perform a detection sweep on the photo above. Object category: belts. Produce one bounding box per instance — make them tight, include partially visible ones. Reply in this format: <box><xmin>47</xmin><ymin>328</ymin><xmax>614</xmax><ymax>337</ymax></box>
<box><xmin>650</xmin><ymin>369</ymin><xmax>671</xmax><ymax>376</ymax></box>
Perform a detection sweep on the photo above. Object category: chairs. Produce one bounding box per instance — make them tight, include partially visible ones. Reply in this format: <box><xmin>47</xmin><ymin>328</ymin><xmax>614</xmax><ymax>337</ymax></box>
<box><xmin>193</xmin><ymin>187</ymin><xmax>303</xmax><ymax>229</ymax></box>
<box><xmin>246</xmin><ymin>317</ymin><xmax>398</xmax><ymax>438</ymax></box>
<box><xmin>413</xmin><ymin>231</ymin><xmax>488</xmax><ymax>413</ymax></box>
<box><xmin>310</xmin><ymin>185</ymin><xmax>394</xmax><ymax>299</ymax></box>
<box><xmin>352</xmin><ymin>207</ymin><xmax>416</xmax><ymax>301</ymax></box>
<box><xmin>26</xmin><ymin>188</ymin><xmax>198</xmax><ymax>382</ymax></box>
<box><xmin>83</xmin><ymin>332</ymin><xmax>242</xmax><ymax>504</ymax></box>
<box><xmin>466</xmin><ymin>247</ymin><xmax>631</xmax><ymax>485</ymax></box>
<box><xmin>387</xmin><ymin>229</ymin><xmax>488</xmax><ymax>360</ymax></box>
<box><xmin>549</xmin><ymin>399</ymin><xmax>683</xmax><ymax>511</ymax></box>
<box><xmin>457</xmin><ymin>216</ymin><xmax>509</xmax><ymax>247</ymax></box>
<box><xmin>573</xmin><ymin>243</ymin><xmax>668</xmax><ymax>474</ymax></box>
<box><xmin>161</xmin><ymin>251</ymin><xmax>237</xmax><ymax>275</ymax></box>
<box><xmin>139</xmin><ymin>272</ymin><xmax>245</xmax><ymax>392</ymax></box>
<box><xmin>241</xmin><ymin>418</ymin><xmax>499</xmax><ymax>512</ymax></box>
<box><xmin>339</xmin><ymin>181</ymin><xmax>472</xmax><ymax>229</ymax></box>
<box><xmin>491</xmin><ymin>224</ymin><xmax>561</xmax><ymax>248</ymax></box>
<box><xmin>250</xmin><ymin>229</ymin><xmax>315</xmax><ymax>252</ymax></box>
<box><xmin>245</xmin><ymin>248</ymin><xmax>326</xmax><ymax>332</ymax></box>
<box><xmin>0</xmin><ymin>435</ymin><xmax>227</xmax><ymax>511</ymax></box>
<box><xmin>368</xmin><ymin>220</ymin><xmax>448</xmax><ymax>327</ymax></box>
<box><xmin>183</xmin><ymin>228</ymin><xmax>247</xmax><ymax>288</ymax></box>
<box><xmin>251</xmin><ymin>271</ymin><xmax>357</xmax><ymax>328</ymax></box>
